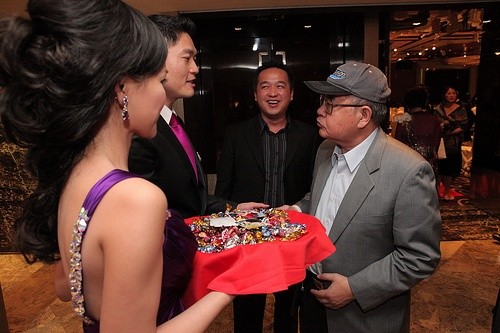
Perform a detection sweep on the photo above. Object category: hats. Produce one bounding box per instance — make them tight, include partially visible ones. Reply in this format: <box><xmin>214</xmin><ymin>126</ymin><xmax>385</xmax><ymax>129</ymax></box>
<box><xmin>304</xmin><ymin>62</ymin><xmax>391</xmax><ymax>104</ymax></box>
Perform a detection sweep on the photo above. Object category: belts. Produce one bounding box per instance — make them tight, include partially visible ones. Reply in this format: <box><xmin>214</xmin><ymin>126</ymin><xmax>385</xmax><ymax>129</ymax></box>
<box><xmin>306</xmin><ymin>273</ymin><xmax>323</xmax><ymax>291</ymax></box>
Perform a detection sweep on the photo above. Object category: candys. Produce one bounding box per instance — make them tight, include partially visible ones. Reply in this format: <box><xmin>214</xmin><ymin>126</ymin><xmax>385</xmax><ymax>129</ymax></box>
<box><xmin>190</xmin><ymin>203</ymin><xmax>309</xmax><ymax>253</ymax></box>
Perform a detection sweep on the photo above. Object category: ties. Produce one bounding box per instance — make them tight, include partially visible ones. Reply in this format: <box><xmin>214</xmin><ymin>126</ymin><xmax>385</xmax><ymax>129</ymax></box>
<box><xmin>170</xmin><ymin>112</ymin><xmax>198</xmax><ymax>182</ymax></box>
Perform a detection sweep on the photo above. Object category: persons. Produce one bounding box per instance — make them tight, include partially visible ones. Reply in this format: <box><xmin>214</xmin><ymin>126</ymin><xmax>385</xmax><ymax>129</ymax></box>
<box><xmin>395</xmin><ymin>86</ymin><xmax>444</xmax><ymax>180</ymax></box>
<box><xmin>215</xmin><ymin>61</ymin><xmax>315</xmax><ymax>333</ymax></box>
<box><xmin>433</xmin><ymin>86</ymin><xmax>476</xmax><ymax>178</ymax></box>
<box><xmin>0</xmin><ymin>0</ymin><xmax>236</xmax><ymax>333</ymax></box>
<box><xmin>128</xmin><ymin>15</ymin><xmax>229</xmax><ymax>216</ymax></box>
<box><xmin>274</xmin><ymin>62</ymin><xmax>441</xmax><ymax>333</ymax></box>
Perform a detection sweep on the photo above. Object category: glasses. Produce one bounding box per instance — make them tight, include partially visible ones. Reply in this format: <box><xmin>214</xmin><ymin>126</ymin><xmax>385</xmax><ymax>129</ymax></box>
<box><xmin>319</xmin><ymin>95</ymin><xmax>362</xmax><ymax>115</ymax></box>
